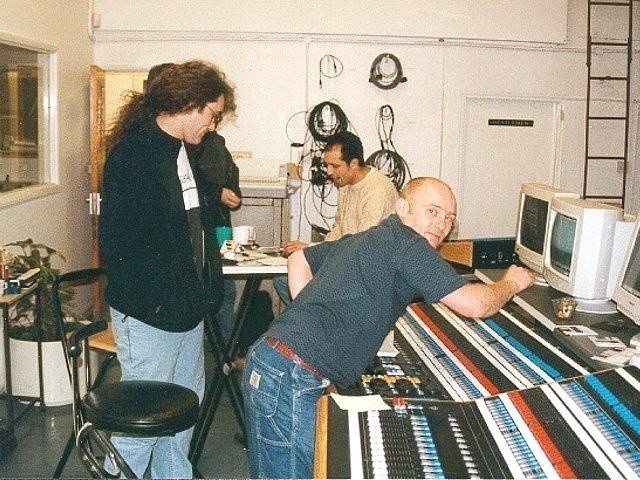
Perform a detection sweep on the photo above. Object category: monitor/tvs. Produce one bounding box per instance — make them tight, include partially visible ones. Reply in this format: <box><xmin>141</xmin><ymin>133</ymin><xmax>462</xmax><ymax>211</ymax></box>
<box><xmin>542</xmin><ymin>198</ymin><xmax>637</xmax><ymax>315</ymax></box>
<box><xmin>611</xmin><ymin>213</ymin><xmax>640</xmax><ymax>351</ymax></box>
<box><xmin>514</xmin><ymin>182</ymin><xmax>581</xmax><ymax>287</ymax></box>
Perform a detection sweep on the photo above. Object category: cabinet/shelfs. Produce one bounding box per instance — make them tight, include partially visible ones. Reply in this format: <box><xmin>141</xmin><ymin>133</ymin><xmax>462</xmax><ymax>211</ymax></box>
<box><xmin>226</xmin><ymin>177</ymin><xmax>286</xmax><ymax>250</ymax></box>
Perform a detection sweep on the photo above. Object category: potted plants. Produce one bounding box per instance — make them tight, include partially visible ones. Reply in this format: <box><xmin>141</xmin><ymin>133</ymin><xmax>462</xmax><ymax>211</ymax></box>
<box><xmin>2</xmin><ymin>239</ymin><xmax>99</xmax><ymax>408</ymax></box>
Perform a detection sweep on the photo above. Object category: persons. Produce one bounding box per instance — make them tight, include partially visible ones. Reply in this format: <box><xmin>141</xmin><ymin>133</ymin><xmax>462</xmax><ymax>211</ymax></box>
<box><xmin>271</xmin><ymin>131</ymin><xmax>400</xmax><ymax>310</ymax></box>
<box><xmin>100</xmin><ymin>61</ymin><xmax>241</xmax><ymax>479</ymax></box>
<box><xmin>239</xmin><ymin>176</ymin><xmax>535</xmax><ymax>478</ymax></box>
<box><xmin>147</xmin><ymin>62</ymin><xmax>244</xmax><ymax>351</ymax></box>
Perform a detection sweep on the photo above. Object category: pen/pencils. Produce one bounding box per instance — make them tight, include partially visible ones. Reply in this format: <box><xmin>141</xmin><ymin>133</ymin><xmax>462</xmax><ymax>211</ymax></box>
<box><xmin>233</xmin><ymin>251</ymin><xmax>250</xmax><ymax>258</ymax></box>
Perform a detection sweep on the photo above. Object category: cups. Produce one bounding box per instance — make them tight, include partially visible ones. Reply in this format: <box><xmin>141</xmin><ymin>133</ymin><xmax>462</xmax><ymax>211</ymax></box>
<box><xmin>551</xmin><ymin>299</ymin><xmax>578</xmax><ymax>319</ymax></box>
<box><xmin>233</xmin><ymin>226</ymin><xmax>256</xmax><ymax>246</ymax></box>
<box><xmin>215</xmin><ymin>227</ymin><xmax>232</xmax><ymax>247</ymax></box>
<box><xmin>0</xmin><ymin>281</ymin><xmax>7</xmax><ymax>296</ymax></box>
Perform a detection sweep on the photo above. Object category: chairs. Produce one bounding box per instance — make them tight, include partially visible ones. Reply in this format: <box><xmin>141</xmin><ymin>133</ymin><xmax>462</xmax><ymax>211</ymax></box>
<box><xmin>51</xmin><ymin>268</ymin><xmax>209</xmax><ymax>479</ymax></box>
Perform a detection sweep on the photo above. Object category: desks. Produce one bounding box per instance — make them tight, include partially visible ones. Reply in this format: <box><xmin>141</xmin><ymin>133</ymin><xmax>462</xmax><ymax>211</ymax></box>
<box><xmin>0</xmin><ymin>279</ymin><xmax>43</xmax><ymax>438</ymax></box>
<box><xmin>185</xmin><ymin>246</ymin><xmax>290</xmax><ymax>479</ymax></box>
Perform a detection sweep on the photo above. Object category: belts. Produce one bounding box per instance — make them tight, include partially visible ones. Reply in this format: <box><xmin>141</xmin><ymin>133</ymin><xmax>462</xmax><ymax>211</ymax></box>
<box><xmin>265</xmin><ymin>335</ymin><xmax>324</xmax><ymax>380</ymax></box>
